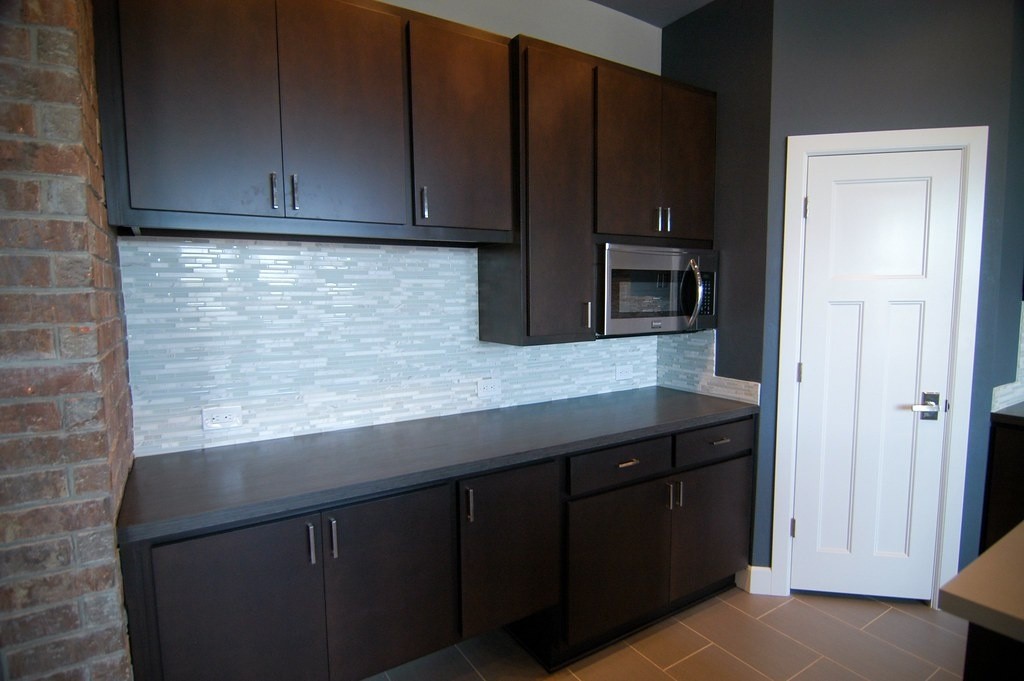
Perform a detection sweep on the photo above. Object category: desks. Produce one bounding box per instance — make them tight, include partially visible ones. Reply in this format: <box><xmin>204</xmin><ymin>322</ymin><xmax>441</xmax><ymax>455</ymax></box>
<box><xmin>939</xmin><ymin>523</ymin><xmax>1024</xmax><ymax>681</ymax></box>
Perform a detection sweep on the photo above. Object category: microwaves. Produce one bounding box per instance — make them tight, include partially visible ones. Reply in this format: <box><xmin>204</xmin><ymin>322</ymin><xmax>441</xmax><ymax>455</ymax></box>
<box><xmin>604</xmin><ymin>243</ymin><xmax>718</xmax><ymax>335</ymax></box>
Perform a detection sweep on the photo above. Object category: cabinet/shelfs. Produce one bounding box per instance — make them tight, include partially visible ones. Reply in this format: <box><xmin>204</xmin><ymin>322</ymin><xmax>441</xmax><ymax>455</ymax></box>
<box><xmin>118</xmin><ymin>417</ymin><xmax>759</xmax><ymax>681</ymax></box>
<box><xmin>95</xmin><ymin>0</ymin><xmax>717</xmax><ymax>348</ymax></box>
